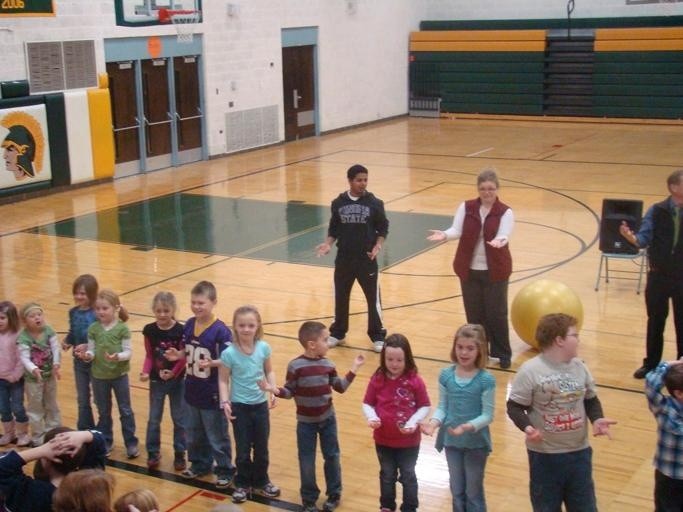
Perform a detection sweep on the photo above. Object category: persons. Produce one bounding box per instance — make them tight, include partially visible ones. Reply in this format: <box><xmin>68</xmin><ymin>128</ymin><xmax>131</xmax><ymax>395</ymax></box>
<box><xmin>164</xmin><ymin>281</ymin><xmax>234</xmax><ymax>490</ymax></box>
<box><xmin>257</xmin><ymin>321</ymin><xmax>365</xmax><ymax>512</ymax></box>
<box><xmin>53</xmin><ymin>471</ymin><xmax>110</xmax><ymax>511</ymax></box>
<box><xmin>62</xmin><ymin>274</ymin><xmax>97</xmax><ymax>432</ymax></box>
<box><xmin>114</xmin><ymin>492</ymin><xmax>159</xmax><ymax>512</ymax></box>
<box><xmin>361</xmin><ymin>334</ymin><xmax>430</xmax><ymax>512</ymax></box>
<box><xmin>426</xmin><ymin>169</ymin><xmax>515</xmax><ymax>369</ymax></box>
<box><xmin>0</xmin><ymin>427</ymin><xmax>106</xmax><ymax>512</ymax></box>
<box><xmin>507</xmin><ymin>313</ymin><xmax>617</xmax><ymax>512</ymax></box>
<box><xmin>644</xmin><ymin>357</ymin><xmax>682</xmax><ymax>512</ymax></box>
<box><xmin>314</xmin><ymin>164</ymin><xmax>390</xmax><ymax>354</ymax></box>
<box><xmin>217</xmin><ymin>308</ymin><xmax>281</xmax><ymax>502</ymax></box>
<box><xmin>618</xmin><ymin>170</ymin><xmax>683</xmax><ymax>379</ymax></box>
<box><xmin>82</xmin><ymin>291</ymin><xmax>139</xmax><ymax>461</ymax></box>
<box><xmin>16</xmin><ymin>301</ymin><xmax>63</xmax><ymax>448</ymax></box>
<box><xmin>419</xmin><ymin>324</ymin><xmax>496</xmax><ymax>512</ymax></box>
<box><xmin>139</xmin><ymin>290</ymin><xmax>187</xmax><ymax>472</ymax></box>
<box><xmin>0</xmin><ymin>111</ymin><xmax>46</xmax><ymax>180</ymax></box>
<box><xmin>0</xmin><ymin>301</ymin><xmax>30</xmax><ymax>446</ymax></box>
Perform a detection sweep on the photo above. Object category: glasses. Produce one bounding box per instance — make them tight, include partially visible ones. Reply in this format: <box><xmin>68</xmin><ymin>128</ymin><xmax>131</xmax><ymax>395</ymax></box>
<box><xmin>565</xmin><ymin>333</ymin><xmax>578</xmax><ymax>339</ymax></box>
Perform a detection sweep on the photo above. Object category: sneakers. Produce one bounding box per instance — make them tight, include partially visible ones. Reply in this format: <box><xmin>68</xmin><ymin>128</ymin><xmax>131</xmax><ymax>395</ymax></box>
<box><xmin>500</xmin><ymin>358</ymin><xmax>511</xmax><ymax>369</ymax></box>
<box><xmin>327</xmin><ymin>336</ymin><xmax>346</xmax><ymax>348</ymax></box>
<box><xmin>374</xmin><ymin>341</ymin><xmax>385</xmax><ymax>353</ymax></box>
<box><xmin>633</xmin><ymin>366</ymin><xmax>652</xmax><ymax>379</ymax></box>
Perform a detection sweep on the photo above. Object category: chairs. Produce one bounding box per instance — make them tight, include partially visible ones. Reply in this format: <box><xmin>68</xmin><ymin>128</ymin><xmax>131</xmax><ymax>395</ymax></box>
<box><xmin>594</xmin><ymin>199</ymin><xmax>648</xmax><ymax>294</ymax></box>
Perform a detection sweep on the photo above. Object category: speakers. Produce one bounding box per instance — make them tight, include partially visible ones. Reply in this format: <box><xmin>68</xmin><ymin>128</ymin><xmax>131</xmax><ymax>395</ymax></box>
<box><xmin>599</xmin><ymin>198</ymin><xmax>644</xmax><ymax>255</ymax></box>
